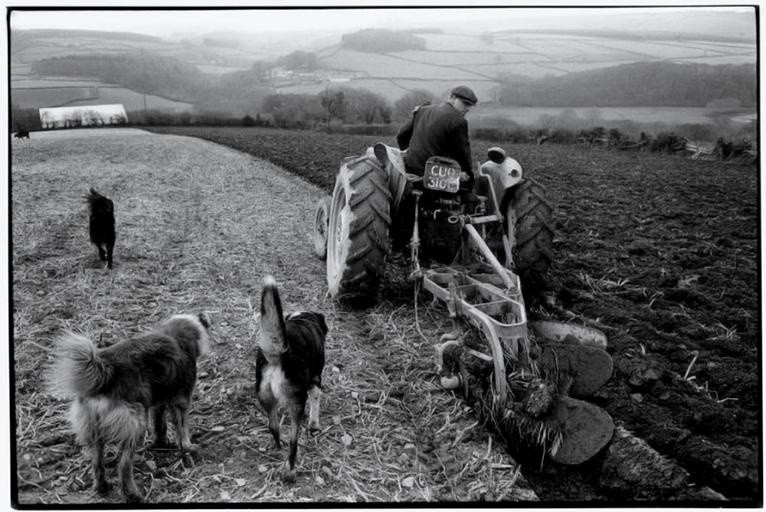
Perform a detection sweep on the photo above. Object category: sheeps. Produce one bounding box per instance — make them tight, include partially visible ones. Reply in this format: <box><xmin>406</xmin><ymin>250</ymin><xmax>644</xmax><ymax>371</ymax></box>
<box><xmin>13</xmin><ymin>129</ymin><xmax>30</xmax><ymax>140</ymax></box>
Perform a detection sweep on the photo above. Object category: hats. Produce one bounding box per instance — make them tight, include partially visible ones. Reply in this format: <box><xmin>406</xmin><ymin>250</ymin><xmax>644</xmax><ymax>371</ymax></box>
<box><xmin>451</xmin><ymin>85</ymin><xmax>477</xmax><ymax>106</ymax></box>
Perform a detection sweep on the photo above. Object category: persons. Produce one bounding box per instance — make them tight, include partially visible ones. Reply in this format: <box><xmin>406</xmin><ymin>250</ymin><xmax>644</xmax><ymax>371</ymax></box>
<box><xmin>394</xmin><ymin>85</ymin><xmax>477</xmax><ymax>192</ymax></box>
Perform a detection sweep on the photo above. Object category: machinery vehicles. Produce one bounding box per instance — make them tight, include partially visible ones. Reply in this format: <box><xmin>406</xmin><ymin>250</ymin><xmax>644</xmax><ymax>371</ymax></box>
<box><xmin>306</xmin><ymin>127</ymin><xmax>620</xmax><ymax>483</ymax></box>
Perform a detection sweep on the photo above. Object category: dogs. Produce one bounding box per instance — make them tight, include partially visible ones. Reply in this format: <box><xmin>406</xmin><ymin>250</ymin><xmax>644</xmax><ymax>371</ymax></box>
<box><xmin>81</xmin><ymin>188</ymin><xmax>116</xmax><ymax>270</ymax></box>
<box><xmin>253</xmin><ymin>274</ymin><xmax>330</xmax><ymax>482</ymax></box>
<box><xmin>46</xmin><ymin>314</ymin><xmax>211</xmax><ymax>505</ymax></box>
